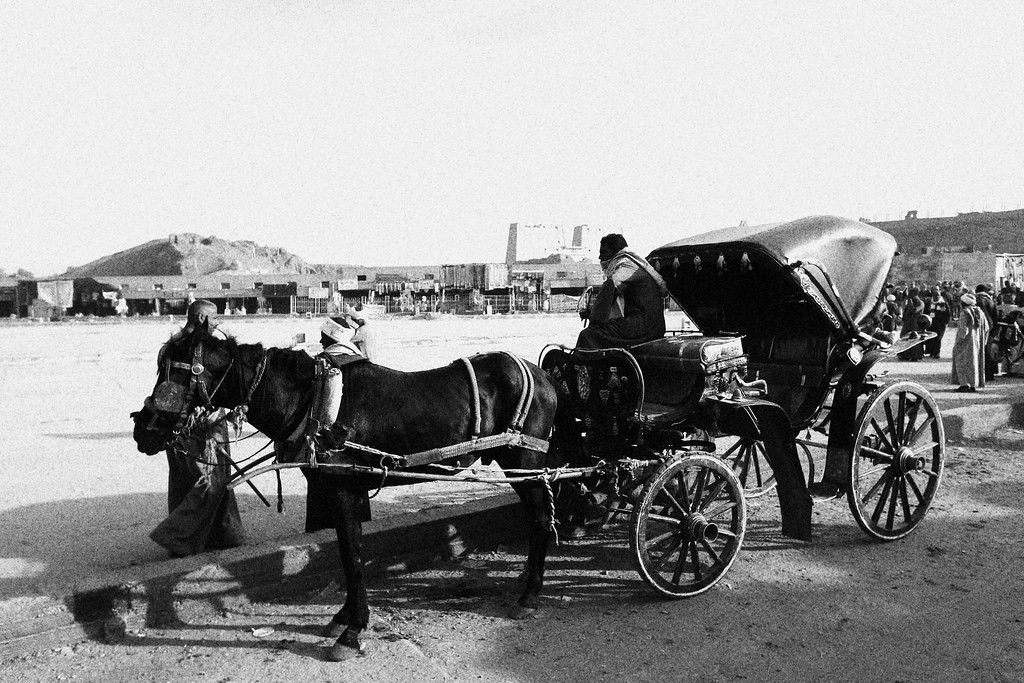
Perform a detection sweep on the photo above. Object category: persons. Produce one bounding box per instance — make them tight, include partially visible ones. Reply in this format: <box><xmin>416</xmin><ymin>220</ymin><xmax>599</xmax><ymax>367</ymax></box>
<box><xmin>574</xmin><ymin>234</ymin><xmax>666</xmax><ymax>351</ymax></box>
<box><xmin>149</xmin><ymin>300</ymin><xmax>371</xmax><ymax>556</ymax></box>
<box><xmin>870</xmin><ymin>281</ymin><xmax>1024</xmax><ymax>393</ymax></box>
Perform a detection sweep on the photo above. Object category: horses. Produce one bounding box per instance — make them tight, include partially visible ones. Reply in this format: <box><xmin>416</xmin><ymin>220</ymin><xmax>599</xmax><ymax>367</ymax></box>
<box><xmin>129</xmin><ymin>316</ymin><xmax>585</xmax><ymax>662</ymax></box>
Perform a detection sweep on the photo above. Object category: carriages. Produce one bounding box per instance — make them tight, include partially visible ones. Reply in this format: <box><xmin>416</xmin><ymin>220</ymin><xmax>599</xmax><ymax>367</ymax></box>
<box><xmin>133</xmin><ymin>216</ymin><xmax>950</xmax><ymax>662</ymax></box>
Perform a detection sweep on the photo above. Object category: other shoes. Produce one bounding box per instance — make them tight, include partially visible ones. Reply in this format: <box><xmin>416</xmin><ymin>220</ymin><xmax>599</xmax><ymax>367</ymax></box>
<box><xmin>899</xmin><ymin>356</ymin><xmax>919</xmax><ymax>362</ymax></box>
<box><xmin>954</xmin><ymin>385</ymin><xmax>975</xmax><ymax>392</ymax></box>
<box><xmin>929</xmin><ymin>354</ymin><xmax>940</xmax><ymax>358</ymax></box>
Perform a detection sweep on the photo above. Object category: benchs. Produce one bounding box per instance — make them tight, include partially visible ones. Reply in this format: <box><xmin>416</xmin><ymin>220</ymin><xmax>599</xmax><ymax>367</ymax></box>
<box><xmin>723</xmin><ymin>312</ymin><xmax>828</xmax><ymax>410</ymax></box>
<box><xmin>616</xmin><ymin>332</ymin><xmax>744</xmax><ymax>382</ymax></box>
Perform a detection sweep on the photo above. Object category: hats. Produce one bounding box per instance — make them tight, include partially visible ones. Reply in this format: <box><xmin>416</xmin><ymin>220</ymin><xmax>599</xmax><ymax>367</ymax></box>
<box><xmin>960</xmin><ymin>293</ymin><xmax>977</xmax><ymax>305</ymax></box>
